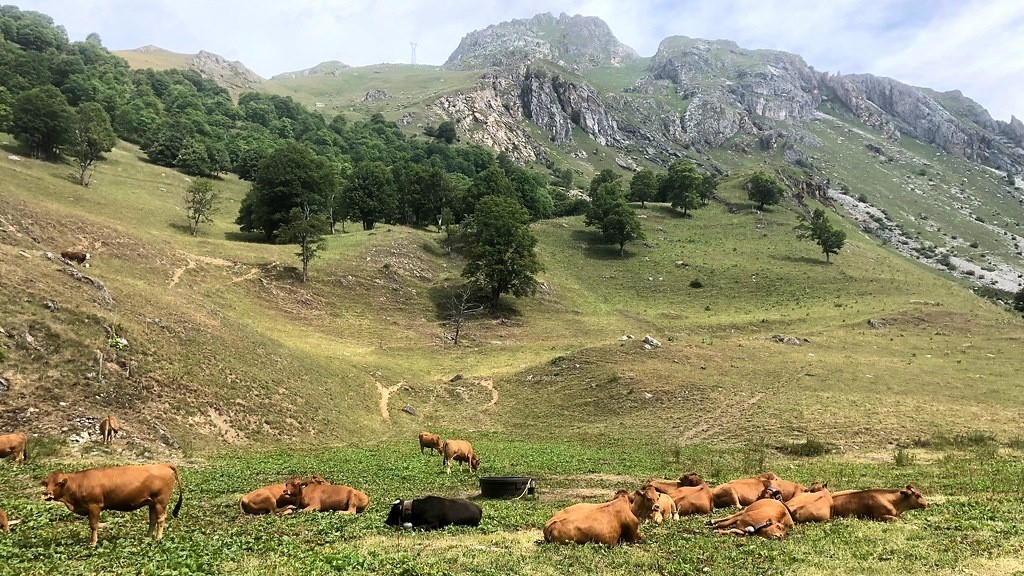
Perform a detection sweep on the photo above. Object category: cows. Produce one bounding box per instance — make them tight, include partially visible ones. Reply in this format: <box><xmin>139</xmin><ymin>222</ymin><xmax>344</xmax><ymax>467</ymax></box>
<box><xmin>442</xmin><ymin>439</ymin><xmax>481</xmax><ymax>476</ymax></box>
<box><xmin>60</xmin><ymin>249</ymin><xmax>92</xmax><ymax>267</ymax></box>
<box><xmin>384</xmin><ymin>494</ymin><xmax>484</xmax><ymax>533</ymax></box>
<box><xmin>238</xmin><ymin>473</ymin><xmax>371</xmax><ymax>518</ymax></box>
<box><xmin>542</xmin><ymin>471</ymin><xmax>929</xmax><ymax>548</ymax></box>
<box><xmin>40</xmin><ymin>462</ymin><xmax>183</xmax><ymax>547</ymax></box>
<box><xmin>0</xmin><ymin>507</ymin><xmax>10</xmax><ymax>535</ymax></box>
<box><xmin>0</xmin><ymin>433</ymin><xmax>30</xmax><ymax>471</ymax></box>
<box><xmin>99</xmin><ymin>415</ymin><xmax>119</xmax><ymax>446</ymax></box>
<box><xmin>418</xmin><ymin>431</ymin><xmax>444</xmax><ymax>457</ymax></box>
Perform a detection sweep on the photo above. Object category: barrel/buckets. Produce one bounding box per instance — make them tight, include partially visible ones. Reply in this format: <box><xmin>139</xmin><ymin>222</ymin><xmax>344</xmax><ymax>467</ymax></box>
<box><xmin>480</xmin><ymin>476</ymin><xmax>536</xmax><ymax>497</ymax></box>
